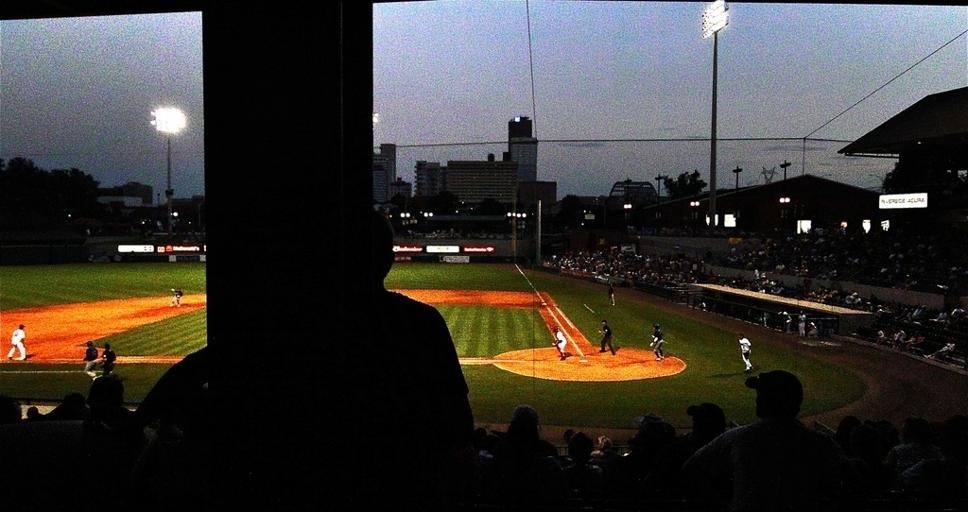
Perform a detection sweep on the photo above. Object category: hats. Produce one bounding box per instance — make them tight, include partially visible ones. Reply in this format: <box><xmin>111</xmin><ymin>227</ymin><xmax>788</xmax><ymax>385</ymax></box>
<box><xmin>686</xmin><ymin>403</ymin><xmax>724</xmax><ymax>420</ymax></box>
<box><xmin>744</xmin><ymin>370</ymin><xmax>803</xmax><ymax>402</ymax></box>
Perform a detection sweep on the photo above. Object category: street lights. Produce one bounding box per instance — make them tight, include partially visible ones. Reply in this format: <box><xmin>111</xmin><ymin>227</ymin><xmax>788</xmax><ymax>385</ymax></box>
<box><xmin>699</xmin><ymin>0</ymin><xmax>730</xmax><ymax>236</ymax></box>
<box><xmin>146</xmin><ymin>104</ymin><xmax>190</xmax><ymax>229</ymax></box>
